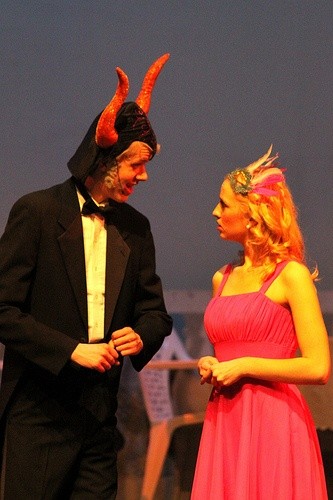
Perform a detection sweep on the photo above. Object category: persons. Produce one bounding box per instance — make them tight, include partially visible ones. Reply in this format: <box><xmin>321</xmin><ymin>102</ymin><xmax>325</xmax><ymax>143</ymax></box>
<box><xmin>187</xmin><ymin>143</ymin><xmax>331</xmax><ymax>500</ymax></box>
<box><xmin>0</xmin><ymin>102</ymin><xmax>176</xmax><ymax>500</ymax></box>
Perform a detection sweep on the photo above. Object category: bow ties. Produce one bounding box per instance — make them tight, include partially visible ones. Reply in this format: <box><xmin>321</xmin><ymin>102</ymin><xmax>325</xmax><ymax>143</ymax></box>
<box><xmin>79</xmin><ymin>185</ymin><xmax>114</xmax><ymax>220</ymax></box>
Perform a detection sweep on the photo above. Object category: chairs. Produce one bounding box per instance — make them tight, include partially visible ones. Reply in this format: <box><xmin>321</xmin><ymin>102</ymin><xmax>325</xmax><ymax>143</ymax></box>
<box><xmin>112</xmin><ymin>309</ymin><xmax>216</xmax><ymax>500</ymax></box>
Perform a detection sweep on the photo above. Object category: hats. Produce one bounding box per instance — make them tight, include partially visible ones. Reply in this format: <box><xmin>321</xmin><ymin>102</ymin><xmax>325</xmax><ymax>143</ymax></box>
<box><xmin>66</xmin><ymin>52</ymin><xmax>175</xmax><ymax>189</ymax></box>
